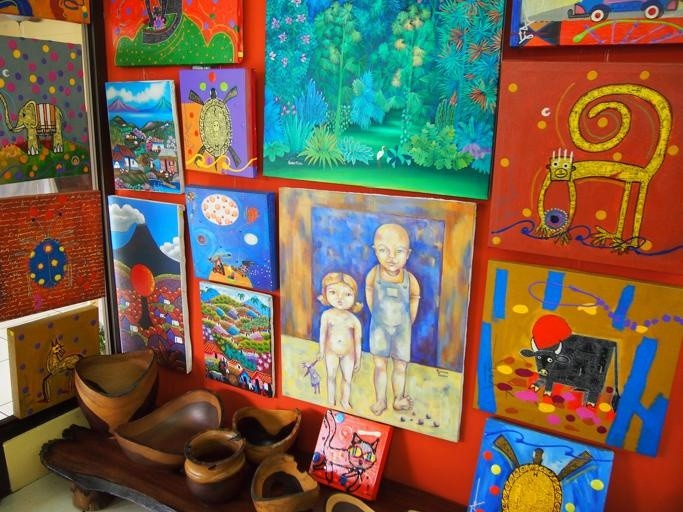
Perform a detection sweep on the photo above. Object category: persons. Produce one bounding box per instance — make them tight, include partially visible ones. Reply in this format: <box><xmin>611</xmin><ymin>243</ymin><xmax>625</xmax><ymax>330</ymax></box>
<box><xmin>317</xmin><ymin>271</ymin><xmax>364</xmax><ymax>412</ymax></box>
<box><xmin>365</xmin><ymin>224</ymin><xmax>422</xmax><ymax>416</ymax></box>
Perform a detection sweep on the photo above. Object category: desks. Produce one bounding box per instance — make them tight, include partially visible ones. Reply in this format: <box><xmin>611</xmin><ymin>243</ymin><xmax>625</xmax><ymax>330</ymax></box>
<box><xmin>40</xmin><ymin>424</ymin><xmax>468</xmax><ymax>512</ymax></box>
